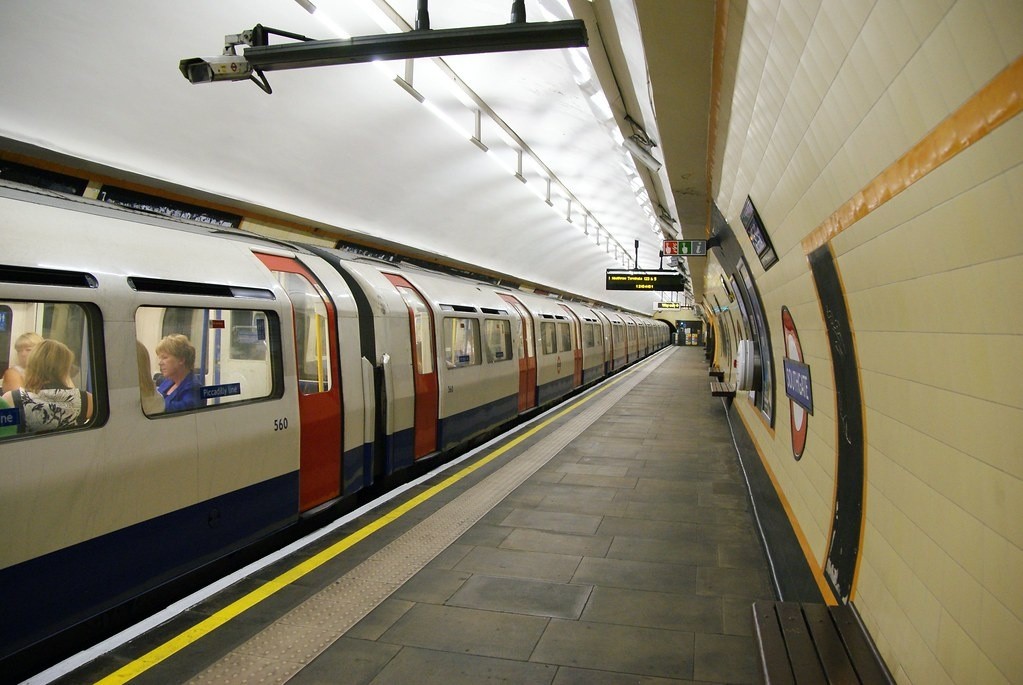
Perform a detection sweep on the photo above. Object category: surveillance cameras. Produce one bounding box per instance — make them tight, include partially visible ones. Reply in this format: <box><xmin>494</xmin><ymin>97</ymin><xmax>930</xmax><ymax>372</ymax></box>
<box><xmin>179</xmin><ymin>55</ymin><xmax>253</xmax><ymax>84</ymax></box>
<box><xmin>622</xmin><ymin>136</ymin><xmax>662</xmax><ymax>173</ymax></box>
<box><xmin>656</xmin><ymin>215</ymin><xmax>679</xmax><ymax>237</ymax></box>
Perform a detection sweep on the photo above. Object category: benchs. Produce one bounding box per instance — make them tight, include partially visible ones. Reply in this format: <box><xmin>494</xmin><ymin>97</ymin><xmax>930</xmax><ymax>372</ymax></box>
<box><xmin>708</xmin><ymin>366</ymin><xmax>724</xmax><ymax>379</ymax></box>
<box><xmin>710</xmin><ymin>381</ymin><xmax>736</xmax><ymax>398</ymax></box>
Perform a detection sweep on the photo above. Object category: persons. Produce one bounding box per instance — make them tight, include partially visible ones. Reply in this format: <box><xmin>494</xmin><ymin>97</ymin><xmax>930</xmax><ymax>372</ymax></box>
<box><xmin>135</xmin><ymin>333</ymin><xmax>207</xmax><ymax>414</ymax></box>
<box><xmin>3</xmin><ymin>333</ymin><xmax>94</xmax><ymax>433</ymax></box>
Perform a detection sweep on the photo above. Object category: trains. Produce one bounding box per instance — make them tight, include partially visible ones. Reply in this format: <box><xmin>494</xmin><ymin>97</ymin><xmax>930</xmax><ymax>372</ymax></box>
<box><xmin>0</xmin><ymin>179</ymin><xmax>672</xmax><ymax>666</ymax></box>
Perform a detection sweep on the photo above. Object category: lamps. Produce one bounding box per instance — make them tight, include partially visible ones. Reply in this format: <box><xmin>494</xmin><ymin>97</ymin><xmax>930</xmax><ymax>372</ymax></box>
<box><xmin>544</xmin><ymin>198</ymin><xmax>553</xmax><ymax>206</ymax></box>
<box><xmin>394</xmin><ymin>75</ymin><xmax>425</xmax><ymax>102</ymax></box>
<box><xmin>513</xmin><ymin>172</ymin><xmax>527</xmax><ymax>183</ymax></box>
<box><xmin>295</xmin><ymin>0</ymin><xmax>316</xmax><ymax>14</ymax></box>
<box><xmin>470</xmin><ymin>137</ymin><xmax>489</xmax><ymax>152</ymax></box>
